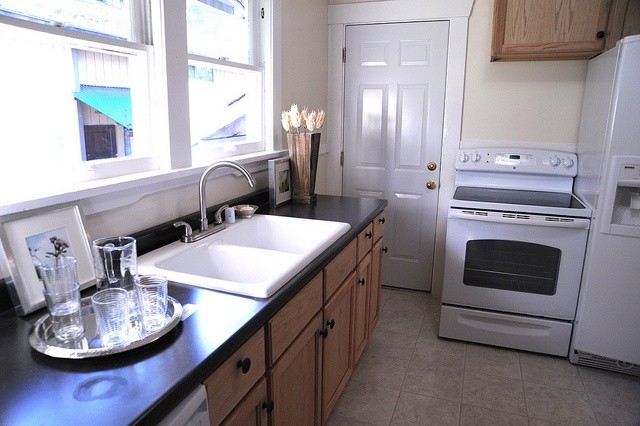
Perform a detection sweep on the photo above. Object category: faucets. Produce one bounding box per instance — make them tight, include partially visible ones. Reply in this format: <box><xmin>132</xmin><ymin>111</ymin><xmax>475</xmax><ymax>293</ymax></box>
<box><xmin>197</xmin><ymin>160</ymin><xmax>256</xmax><ymax>231</ymax></box>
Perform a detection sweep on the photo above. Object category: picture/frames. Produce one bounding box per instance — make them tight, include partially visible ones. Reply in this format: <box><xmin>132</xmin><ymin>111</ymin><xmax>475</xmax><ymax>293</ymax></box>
<box><xmin>0</xmin><ymin>203</ymin><xmax>105</xmax><ymax>314</ymax></box>
<box><xmin>267</xmin><ymin>156</ymin><xmax>292</xmax><ymax>208</ymax></box>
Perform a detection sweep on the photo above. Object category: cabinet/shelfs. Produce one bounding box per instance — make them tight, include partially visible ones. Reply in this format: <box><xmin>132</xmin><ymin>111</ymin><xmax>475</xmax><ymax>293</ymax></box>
<box><xmin>266</xmin><ymin>238</ymin><xmax>356</xmax><ymax>425</ymax></box>
<box><xmin>207</xmin><ymin>326</ymin><xmax>267</xmax><ymax>425</ymax></box>
<box><xmin>492</xmin><ymin>2</ymin><xmax>639</xmax><ymax>63</ymax></box>
<box><xmin>370</xmin><ymin>209</ymin><xmax>386</xmax><ymax>338</ymax></box>
<box><xmin>358</xmin><ymin>225</ymin><xmax>373</xmax><ymax>367</ymax></box>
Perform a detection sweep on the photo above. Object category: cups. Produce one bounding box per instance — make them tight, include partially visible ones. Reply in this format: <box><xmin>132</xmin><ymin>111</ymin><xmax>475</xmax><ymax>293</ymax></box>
<box><xmin>91</xmin><ymin>287</ymin><xmax>130</xmax><ymax>348</ymax></box>
<box><xmin>43</xmin><ymin>283</ymin><xmax>85</xmax><ymax>341</ymax></box>
<box><xmin>134</xmin><ymin>272</ymin><xmax>168</xmax><ymax>332</ymax></box>
<box><xmin>37</xmin><ymin>256</ymin><xmax>80</xmax><ymax>318</ymax></box>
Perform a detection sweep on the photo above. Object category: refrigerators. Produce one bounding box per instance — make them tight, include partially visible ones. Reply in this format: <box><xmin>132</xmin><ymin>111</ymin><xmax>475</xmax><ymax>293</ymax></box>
<box><xmin>569</xmin><ymin>33</ymin><xmax>639</xmax><ymax>379</ymax></box>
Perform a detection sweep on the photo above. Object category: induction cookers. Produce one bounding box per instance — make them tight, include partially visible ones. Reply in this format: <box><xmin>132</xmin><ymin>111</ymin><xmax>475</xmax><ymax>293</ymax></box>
<box><xmin>449</xmin><ymin>185</ymin><xmax>593</xmax><ymax>219</ymax></box>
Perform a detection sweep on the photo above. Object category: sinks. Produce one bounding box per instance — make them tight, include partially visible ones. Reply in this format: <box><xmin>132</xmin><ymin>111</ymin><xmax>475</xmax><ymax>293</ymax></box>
<box><xmin>155</xmin><ymin>245</ymin><xmax>297</xmax><ymax>285</ymax></box>
<box><xmin>223</xmin><ymin>214</ymin><xmax>341</xmax><ymax>253</ymax></box>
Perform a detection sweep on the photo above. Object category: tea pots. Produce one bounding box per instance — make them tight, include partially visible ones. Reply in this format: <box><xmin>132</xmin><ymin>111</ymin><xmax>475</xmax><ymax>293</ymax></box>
<box><xmin>92</xmin><ymin>233</ymin><xmax>138</xmax><ymax>292</ymax></box>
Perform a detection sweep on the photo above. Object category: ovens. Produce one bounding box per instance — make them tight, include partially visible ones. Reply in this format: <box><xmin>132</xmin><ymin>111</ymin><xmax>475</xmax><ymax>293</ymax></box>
<box><xmin>438</xmin><ymin>208</ymin><xmax>591</xmax><ymax>357</ymax></box>
